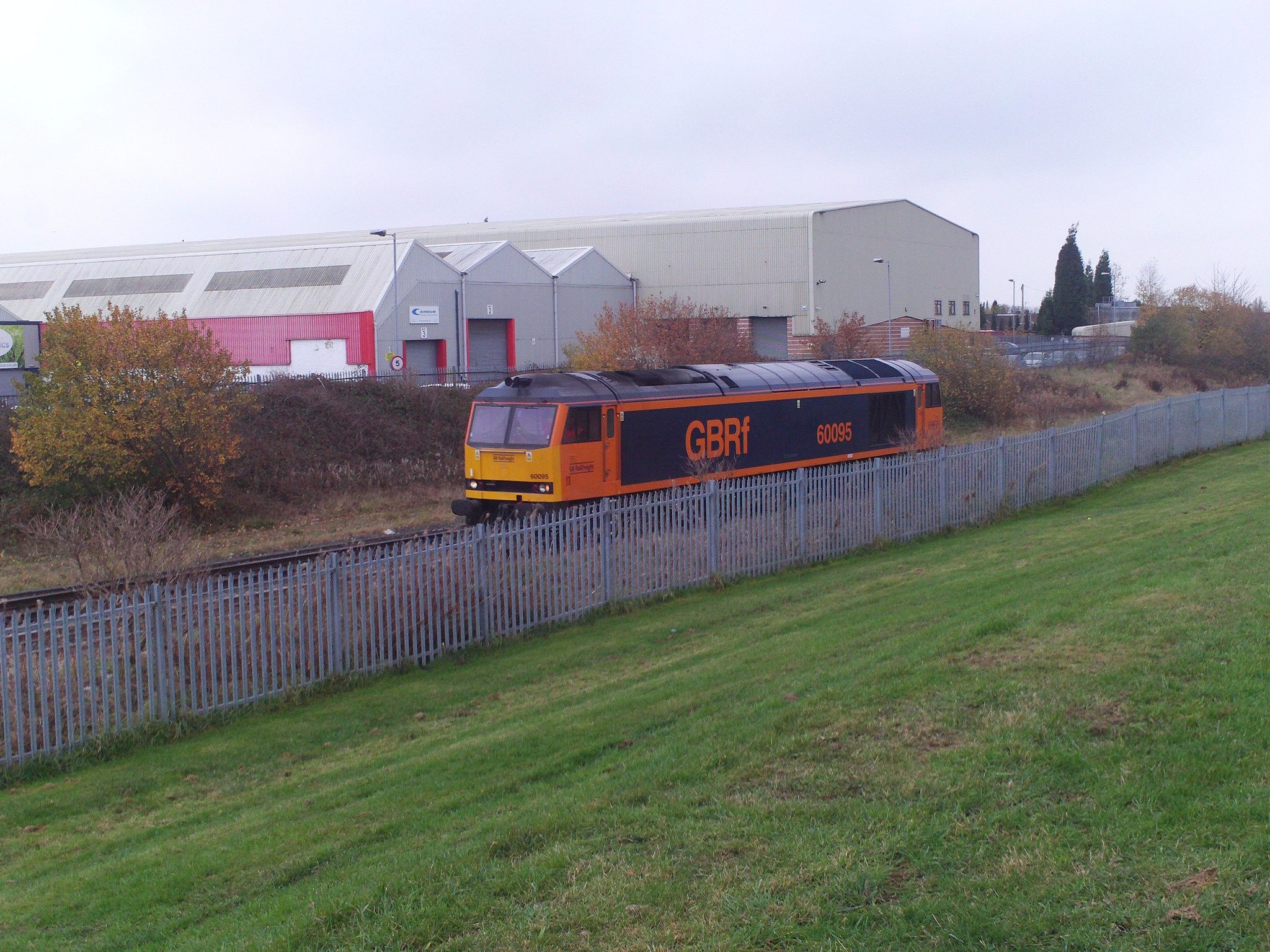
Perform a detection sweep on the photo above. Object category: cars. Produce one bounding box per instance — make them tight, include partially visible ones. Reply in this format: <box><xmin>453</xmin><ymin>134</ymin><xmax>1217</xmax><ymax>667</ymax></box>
<box><xmin>1049</xmin><ymin>351</ymin><xmax>1078</xmax><ymax>366</ymax></box>
<box><xmin>1115</xmin><ymin>347</ymin><xmax>1126</xmax><ymax>356</ymax></box>
<box><xmin>1005</xmin><ymin>355</ymin><xmax>1027</xmax><ymax>366</ymax></box>
<box><xmin>994</xmin><ymin>341</ymin><xmax>1020</xmax><ymax>354</ymax></box>
<box><xmin>1076</xmin><ymin>350</ymin><xmax>1091</xmax><ymax>363</ymax></box>
<box><xmin>1023</xmin><ymin>352</ymin><xmax>1053</xmax><ymax>367</ymax></box>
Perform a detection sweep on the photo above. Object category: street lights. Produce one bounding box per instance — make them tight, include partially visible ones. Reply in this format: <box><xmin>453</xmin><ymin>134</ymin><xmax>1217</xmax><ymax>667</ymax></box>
<box><xmin>1009</xmin><ymin>278</ymin><xmax>1016</xmax><ymax>343</ymax></box>
<box><xmin>873</xmin><ymin>258</ymin><xmax>893</xmax><ymax>356</ymax></box>
<box><xmin>1101</xmin><ymin>272</ymin><xmax>1116</xmax><ymax>343</ymax></box>
<box><xmin>370</xmin><ymin>228</ymin><xmax>399</xmax><ymax>378</ymax></box>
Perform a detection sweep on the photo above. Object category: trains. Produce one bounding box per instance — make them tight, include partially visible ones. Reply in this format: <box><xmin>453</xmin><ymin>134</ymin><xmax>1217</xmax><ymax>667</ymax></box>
<box><xmin>449</xmin><ymin>352</ymin><xmax>947</xmax><ymax>548</ymax></box>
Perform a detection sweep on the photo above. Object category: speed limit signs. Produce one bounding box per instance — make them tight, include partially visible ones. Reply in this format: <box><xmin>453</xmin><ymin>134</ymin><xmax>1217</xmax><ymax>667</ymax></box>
<box><xmin>392</xmin><ymin>356</ymin><xmax>404</xmax><ymax>370</ymax></box>
